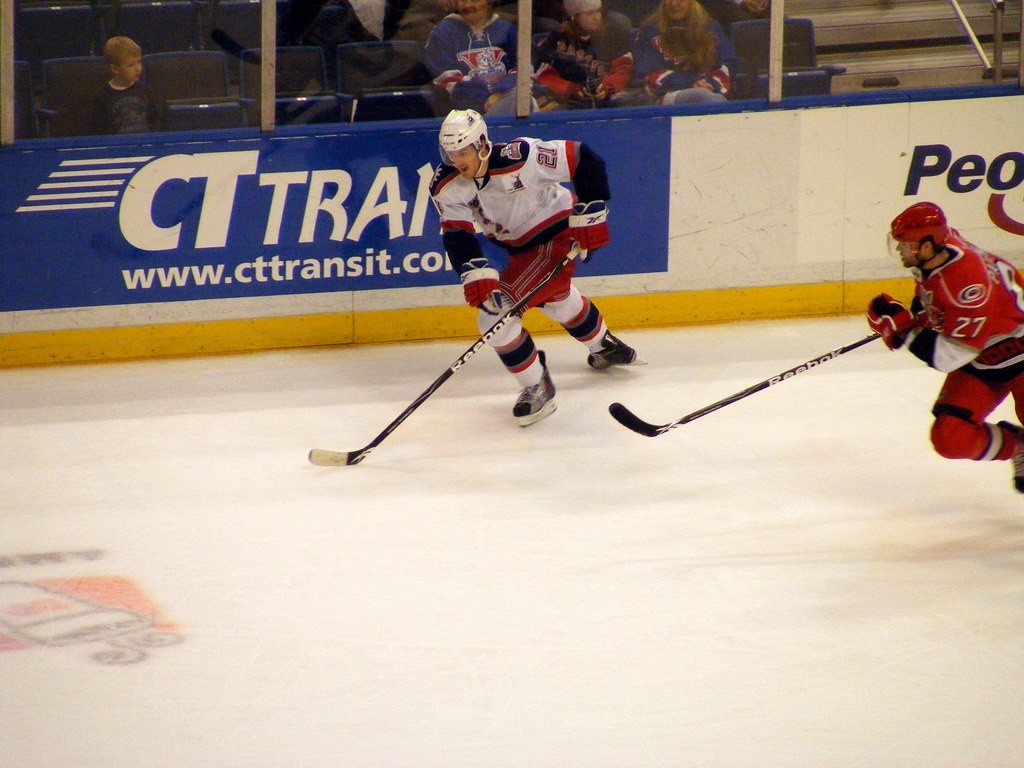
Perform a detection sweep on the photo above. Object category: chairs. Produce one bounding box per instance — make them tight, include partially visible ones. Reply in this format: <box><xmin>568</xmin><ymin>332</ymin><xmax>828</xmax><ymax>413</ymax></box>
<box><xmin>13</xmin><ymin>0</ymin><xmax>849</xmax><ymax>137</ymax></box>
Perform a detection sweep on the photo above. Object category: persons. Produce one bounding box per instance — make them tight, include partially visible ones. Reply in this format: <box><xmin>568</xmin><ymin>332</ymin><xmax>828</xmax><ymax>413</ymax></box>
<box><xmin>429</xmin><ymin>109</ymin><xmax>647</xmax><ymax>429</ymax></box>
<box><xmin>866</xmin><ymin>200</ymin><xmax>1024</xmax><ymax>496</ymax></box>
<box><xmin>90</xmin><ymin>36</ymin><xmax>165</xmax><ymax>135</ymax></box>
<box><xmin>423</xmin><ymin>0</ymin><xmax>789</xmax><ymax>135</ymax></box>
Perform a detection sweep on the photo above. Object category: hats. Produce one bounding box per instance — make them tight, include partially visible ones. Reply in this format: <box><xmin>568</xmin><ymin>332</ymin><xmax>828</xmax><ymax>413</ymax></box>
<box><xmin>564</xmin><ymin>0</ymin><xmax>602</xmax><ymax>15</ymax></box>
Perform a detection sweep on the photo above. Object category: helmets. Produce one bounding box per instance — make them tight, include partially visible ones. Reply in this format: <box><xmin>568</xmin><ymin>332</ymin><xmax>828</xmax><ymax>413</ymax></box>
<box><xmin>439</xmin><ymin>108</ymin><xmax>492</xmax><ymax>160</ymax></box>
<box><xmin>891</xmin><ymin>202</ymin><xmax>947</xmax><ymax>257</ymax></box>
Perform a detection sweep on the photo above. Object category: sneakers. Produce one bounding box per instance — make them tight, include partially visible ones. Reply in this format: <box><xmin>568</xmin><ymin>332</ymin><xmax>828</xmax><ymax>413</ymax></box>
<box><xmin>992</xmin><ymin>421</ymin><xmax>1024</xmax><ymax>493</ymax></box>
<box><xmin>587</xmin><ymin>330</ymin><xmax>648</xmax><ymax>370</ymax></box>
<box><xmin>513</xmin><ymin>351</ymin><xmax>558</xmax><ymax>427</ymax></box>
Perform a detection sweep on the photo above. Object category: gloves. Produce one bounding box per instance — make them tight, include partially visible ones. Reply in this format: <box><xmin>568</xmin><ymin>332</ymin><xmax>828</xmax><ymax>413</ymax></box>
<box><xmin>569</xmin><ymin>200</ymin><xmax>609</xmax><ymax>263</ymax></box>
<box><xmin>460</xmin><ymin>258</ymin><xmax>503</xmax><ymax>315</ymax></box>
<box><xmin>867</xmin><ymin>293</ymin><xmax>914</xmax><ymax>350</ymax></box>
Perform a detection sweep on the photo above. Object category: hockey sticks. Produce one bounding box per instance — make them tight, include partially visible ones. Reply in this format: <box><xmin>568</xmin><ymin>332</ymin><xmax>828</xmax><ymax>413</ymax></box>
<box><xmin>609</xmin><ymin>333</ymin><xmax>882</xmax><ymax>437</ymax></box>
<box><xmin>309</xmin><ymin>245</ymin><xmax>580</xmax><ymax>467</ymax></box>
